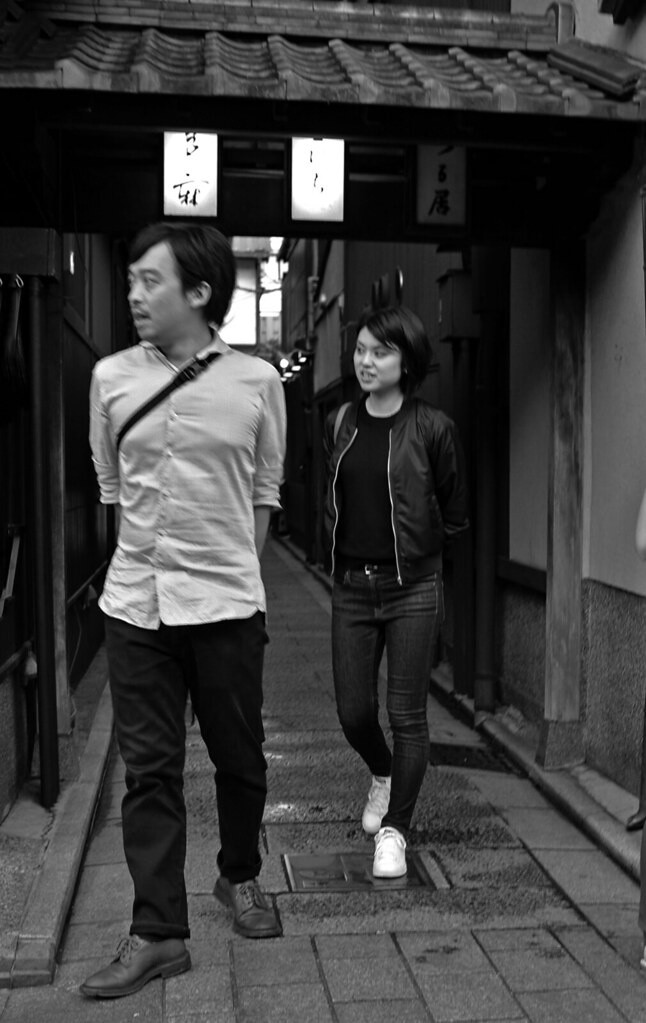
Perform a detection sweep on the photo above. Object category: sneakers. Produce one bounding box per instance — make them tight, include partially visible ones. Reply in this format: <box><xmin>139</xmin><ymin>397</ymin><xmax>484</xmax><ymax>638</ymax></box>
<box><xmin>362</xmin><ymin>776</ymin><xmax>391</xmax><ymax>833</ymax></box>
<box><xmin>374</xmin><ymin>826</ymin><xmax>407</xmax><ymax>878</ymax></box>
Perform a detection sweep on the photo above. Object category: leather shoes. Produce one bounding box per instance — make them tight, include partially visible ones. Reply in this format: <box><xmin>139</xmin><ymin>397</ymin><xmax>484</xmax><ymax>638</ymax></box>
<box><xmin>80</xmin><ymin>934</ymin><xmax>192</xmax><ymax>996</ymax></box>
<box><xmin>213</xmin><ymin>874</ymin><xmax>282</xmax><ymax>938</ymax></box>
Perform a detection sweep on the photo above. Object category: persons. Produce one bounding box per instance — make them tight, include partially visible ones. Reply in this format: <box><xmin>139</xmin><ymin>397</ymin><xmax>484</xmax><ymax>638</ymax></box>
<box><xmin>317</xmin><ymin>306</ymin><xmax>475</xmax><ymax>879</ymax></box>
<box><xmin>78</xmin><ymin>220</ymin><xmax>288</xmax><ymax>998</ymax></box>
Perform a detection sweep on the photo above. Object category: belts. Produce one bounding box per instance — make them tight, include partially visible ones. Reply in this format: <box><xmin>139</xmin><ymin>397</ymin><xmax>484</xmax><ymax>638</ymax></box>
<box><xmin>352</xmin><ymin>563</ymin><xmax>393</xmax><ymax>575</ymax></box>
